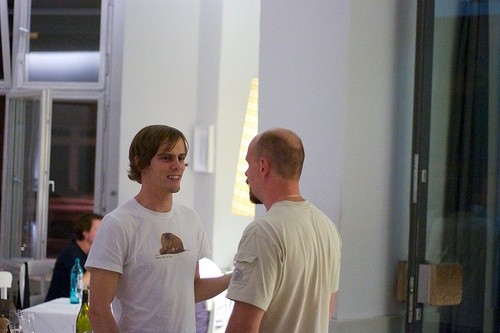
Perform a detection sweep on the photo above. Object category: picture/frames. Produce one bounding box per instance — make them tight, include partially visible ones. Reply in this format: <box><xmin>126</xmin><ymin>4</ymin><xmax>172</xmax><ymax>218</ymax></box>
<box><xmin>192</xmin><ymin>123</ymin><xmax>214</xmax><ymax>174</ymax></box>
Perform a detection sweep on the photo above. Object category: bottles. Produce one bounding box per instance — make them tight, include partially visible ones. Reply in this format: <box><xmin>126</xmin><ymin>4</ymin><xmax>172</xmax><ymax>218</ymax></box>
<box><xmin>70</xmin><ymin>257</ymin><xmax>83</xmax><ymax>304</ymax></box>
<box><xmin>0</xmin><ymin>314</ymin><xmax>8</xmax><ymax>333</ymax></box>
<box><xmin>76</xmin><ymin>290</ymin><xmax>92</xmax><ymax>333</ymax></box>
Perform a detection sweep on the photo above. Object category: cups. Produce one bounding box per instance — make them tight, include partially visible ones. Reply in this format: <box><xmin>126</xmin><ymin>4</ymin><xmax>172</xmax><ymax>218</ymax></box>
<box><xmin>21</xmin><ymin>311</ymin><xmax>36</xmax><ymax>333</ymax></box>
<box><xmin>8</xmin><ymin>308</ymin><xmax>22</xmax><ymax>333</ymax></box>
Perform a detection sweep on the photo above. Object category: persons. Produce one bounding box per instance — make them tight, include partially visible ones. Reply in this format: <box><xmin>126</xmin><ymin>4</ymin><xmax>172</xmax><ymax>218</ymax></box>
<box><xmin>82</xmin><ymin>125</ymin><xmax>232</xmax><ymax>333</ymax></box>
<box><xmin>225</xmin><ymin>128</ymin><xmax>340</xmax><ymax>333</ymax></box>
<box><xmin>44</xmin><ymin>212</ymin><xmax>104</xmax><ymax>302</ymax></box>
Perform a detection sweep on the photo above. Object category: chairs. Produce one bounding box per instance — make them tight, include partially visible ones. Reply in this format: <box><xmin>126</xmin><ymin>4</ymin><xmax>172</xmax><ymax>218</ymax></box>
<box><xmin>18</xmin><ymin>259</ymin><xmax>58</xmax><ymax>303</ymax></box>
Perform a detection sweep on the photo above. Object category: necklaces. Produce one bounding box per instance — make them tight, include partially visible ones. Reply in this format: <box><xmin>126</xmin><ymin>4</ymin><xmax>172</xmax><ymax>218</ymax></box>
<box><xmin>266</xmin><ymin>194</ymin><xmax>302</xmax><ymax>210</ymax></box>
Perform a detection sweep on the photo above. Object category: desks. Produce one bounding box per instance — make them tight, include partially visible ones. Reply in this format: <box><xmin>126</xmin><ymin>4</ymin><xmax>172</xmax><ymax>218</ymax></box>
<box><xmin>20</xmin><ymin>297</ymin><xmax>114</xmax><ymax>333</ymax></box>
